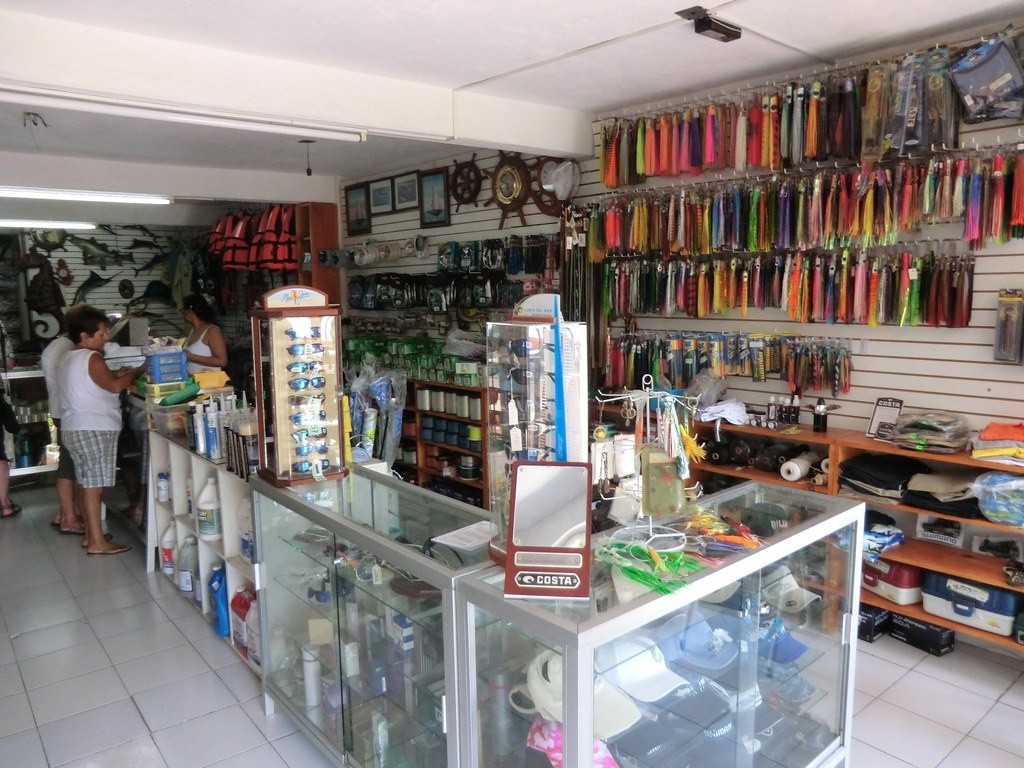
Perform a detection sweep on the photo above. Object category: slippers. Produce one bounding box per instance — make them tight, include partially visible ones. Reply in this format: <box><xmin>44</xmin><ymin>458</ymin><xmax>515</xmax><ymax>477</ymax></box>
<box><xmin>60</xmin><ymin>521</ymin><xmax>85</xmax><ymax>535</ymax></box>
<box><xmin>87</xmin><ymin>545</ymin><xmax>131</xmax><ymax>555</ymax></box>
<box><xmin>81</xmin><ymin>534</ymin><xmax>112</xmax><ymax>547</ymax></box>
<box><xmin>52</xmin><ymin>515</ymin><xmax>62</xmax><ymax>526</ymax></box>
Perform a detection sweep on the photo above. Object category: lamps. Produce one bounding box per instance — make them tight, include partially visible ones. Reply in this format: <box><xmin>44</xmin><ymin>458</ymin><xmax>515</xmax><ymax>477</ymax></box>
<box><xmin>0</xmin><ymin>219</ymin><xmax>99</xmax><ymax>230</ymax></box>
<box><xmin>675</xmin><ymin>6</ymin><xmax>741</xmax><ymax>43</ymax></box>
<box><xmin>0</xmin><ymin>83</ymin><xmax>368</xmax><ymax>142</ymax></box>
<box><xmin>0</xmin><ymin>185</ymin><xmax>175</xmax><ymax>205</ymax></box>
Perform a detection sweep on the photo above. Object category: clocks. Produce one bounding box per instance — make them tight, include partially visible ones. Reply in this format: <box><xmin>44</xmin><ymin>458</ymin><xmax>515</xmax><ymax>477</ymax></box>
<box><xmin>501</xmin><ymin>174</ymin><xmax>514</xmax><ymax>197</ymax></box>
<box><xmin>541</xmin><ymin>161</ymin><xmax>558</xmax><ymax>192</ymax></box>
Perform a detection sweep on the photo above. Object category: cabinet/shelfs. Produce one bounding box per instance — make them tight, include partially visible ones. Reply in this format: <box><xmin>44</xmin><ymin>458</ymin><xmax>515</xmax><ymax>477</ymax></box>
<box><xmin>0</xmin><ymin>354</ymin><xmax>1023</xmax><ymax>768</ymax></box>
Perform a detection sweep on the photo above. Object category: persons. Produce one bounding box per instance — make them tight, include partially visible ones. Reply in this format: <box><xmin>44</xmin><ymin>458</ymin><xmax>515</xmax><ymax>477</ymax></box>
<box><xmin>182</xmin><ymin>294</ymin><xmax>227</xmax><ymax>378</ymax></box>
<box><xmin>41</xmin><ymin>305</ymin><xmax>96</xmax><ymax>535</ymax></box>
<box><xmin>57</xmin><ymin>311</ymin><xmax>146</xmax><ymax>554</ymax></box>
<box><xmin>0</xmin><ymin>319</ymin><xmax>22</xmax><ymax>520</ymax></box>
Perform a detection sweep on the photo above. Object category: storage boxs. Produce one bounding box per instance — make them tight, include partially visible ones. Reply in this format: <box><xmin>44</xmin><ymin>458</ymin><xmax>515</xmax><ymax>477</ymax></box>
<box><xmin>861</xmin><ymin>559</ymin><xmax>922</xmax><ymax>605</ymax></box>
<box><xmin>889</xmin><ymin>612</ymin><xmax>955</xmax><ymax>657</ymax></box>
<box><xmin>921</xmin><ymin>572</ymin><xmax>1020</xmax><ymax>636</ymax></box>
<box><xmin>857</xmin><ymin>603</ymin><xmax>889</xmax><ymax>643</ymax></box>
<box><xmin>1013</xmin><ymin>613</ymin><xmax>1024</xmax><ymax>645</ymax></box>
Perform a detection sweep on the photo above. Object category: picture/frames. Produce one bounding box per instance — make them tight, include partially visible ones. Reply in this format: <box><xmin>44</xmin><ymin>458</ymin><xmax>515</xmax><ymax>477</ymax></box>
<box><xmin>368</xmin><ymin>176</ymin><xmax>394</xmax><ymax>218</ymax></box>
<box><xmin>393</xmin><ymin>170</ymin><xmax>420</xmax><ymax>214</ymax></box>
<box><xmin>344</xmin><ymin>181</ymin><xmax>372</xmax><ymax>237</ymax></box>
<box><xmin>420</xmin><ymin>166</ymin><xmax>451</xmax><ymax>229</ymax></box>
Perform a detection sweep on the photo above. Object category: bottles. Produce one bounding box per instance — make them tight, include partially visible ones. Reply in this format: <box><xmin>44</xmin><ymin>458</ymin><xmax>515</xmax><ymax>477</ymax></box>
<box><xmin>238</xmin><ymin>498</ymin><xmax>255</xmax><ymax>570</ymax></box>
<box><xmin>157</xmin><ymin>473</ymin><xmax>169</xmax><ymax>502</ymax></box>
<box><xmin>197</xmin><ymin>478</ymin><xmax>222</xmax><ymax>542</ymax></box>
<box><xmin>230</xmin><ymin>587</ymin><xmax>261</xmax><ymax>667</ymax></box>
<box><xmin>209</xmin><ymin>562</ymin><xmax>229</xmax><ymax>637</ymax></box>
<box><xmin>160</xmin><ymin>519</ymin><xmax>177</xmax><ymax>574</ymax></box>
<box><xmin>178</xmin><ymin>535</ymin><xmax>202</xmax><ymax>606</ymax></box>
<box><xmin>187</xmin><ymin>391</ymin><xmax>247</xmax><ymax>460</ymax></box>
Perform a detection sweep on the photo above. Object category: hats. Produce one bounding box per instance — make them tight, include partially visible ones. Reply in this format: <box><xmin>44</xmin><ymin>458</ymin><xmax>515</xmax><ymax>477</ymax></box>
<box><xmin>719</xmin><ymin>596</ymin><xmax>810</xmax><ymax>664</ymax></box>
<box><xmin>525</xmin><ymin>654</ymin><xmax>837</xmax><ymax>768</ymax></box>
<box><xmin>651</xmin><ymin>604</ymin><xmax>740</xmax><ymax>671</ymax></box>
<box><xmin>527</xmin><ymin>646</ymin><xmax>641</xmax><ymax>739</ymax></box>
<box><xmin>598</xmin><ymin>637</ymin><xmax>689</xmax><ymax>701</ymax></box>
<box><xmin>757</xmin><ymin>563</ymin><xmax>821</xmax><ymax>614</ymax></box>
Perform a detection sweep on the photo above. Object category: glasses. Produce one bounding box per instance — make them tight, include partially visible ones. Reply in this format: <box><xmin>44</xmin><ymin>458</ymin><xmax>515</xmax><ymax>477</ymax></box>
<box><xmin>292</xmin><ymin>459</ymin><xmax>329</xmax><ymax>473</ymax></box>
<box><xmin>284</xmin><ymin>326</ymin><xmax>322</xmax><ymax>341</ymax></box>
<box><xmin>288</xmin><ymin>409</ymin><xmax>326</xmax><ymax>426</ymax></box>
<box><xmin>483</xmin><ymin>328</ymin><xmax>558</xmax><ymax>465</ymax></box>
<box><xmin>289</xmin><ymin>427</ymin><xmax>327</xmax><ymax>443</ymax></box>
<box><xmin>287</xmin><ymin>361</ymin><xmax>325</xmax><ymax>375</ymax></box>
<box><xmin>293</xmin><ymin>444</ymin><xmax>329</xmax><ymax>456</ymax></box>
<box><xmin>288</xmin><ymin>377</ymin><xmax>327</xmax><ymax>392</ymax></box>
<box><xmin>288</xmin><ymin>393</ymin><xmax>326</xmax><ymax>408</ymax></box>
<box><xmin>305</xmin><ymin>587</ymin><xmax>332</xmax><ymax>602</ymax></box>
<box><xmin>179</xmin><ymin>306</ymin><xmax>193</xmax><ymax>313</ymax></box>
<box><xmin>287</xmin><ymin>343</ymin><xmax>326</xmax><ymax>358</ymax></box>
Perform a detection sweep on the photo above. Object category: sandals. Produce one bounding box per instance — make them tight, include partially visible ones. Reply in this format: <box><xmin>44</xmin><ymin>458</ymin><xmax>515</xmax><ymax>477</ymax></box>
<box><xmin>0</xmin><ymin>499</ymin><xmax>21</xmax><ymax>519</ymax></box>
